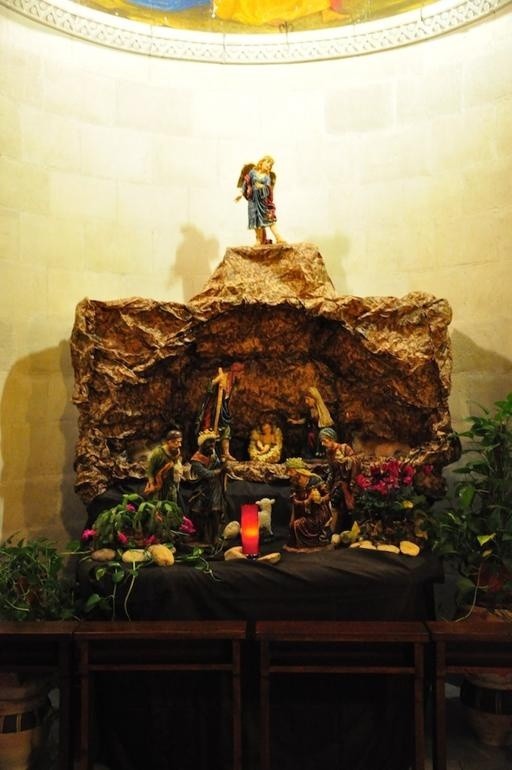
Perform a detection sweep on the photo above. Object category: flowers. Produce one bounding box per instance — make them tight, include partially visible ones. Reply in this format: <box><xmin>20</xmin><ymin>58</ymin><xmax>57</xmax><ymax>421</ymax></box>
<box><xmin>81</xmin><ymin>492</ymin><xmax>197</xmax><ymax>548</ymax></box>
<box><xmin>354</xmin><ymin>459</ymin><xmax>413</xmax><ymax>535</ymax></box>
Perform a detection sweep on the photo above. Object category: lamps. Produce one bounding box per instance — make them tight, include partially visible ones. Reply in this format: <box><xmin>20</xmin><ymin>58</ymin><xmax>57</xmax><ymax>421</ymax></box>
<box><xmin>240</xmin><ymin>504</ymin><xmax>260</xmax><ymax>560</ymax></box>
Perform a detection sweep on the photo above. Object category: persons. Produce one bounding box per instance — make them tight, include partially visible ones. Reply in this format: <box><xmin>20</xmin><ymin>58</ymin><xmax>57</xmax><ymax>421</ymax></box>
<box><xmin>243</xmin><ymin>154</ymin><xmax>288</xmax><ymax>244</ymax></box>
<box><xmin>144</xmin><ymin>385</ymin><xmax>359</xmax><ymax>550</ymax></box>
